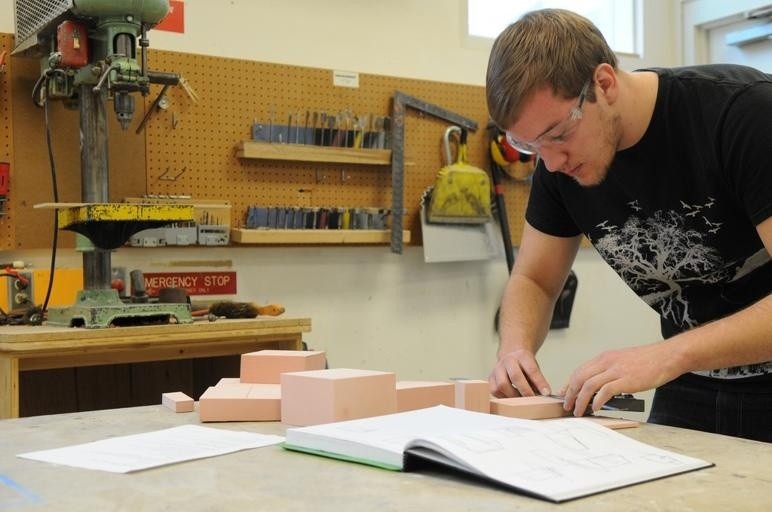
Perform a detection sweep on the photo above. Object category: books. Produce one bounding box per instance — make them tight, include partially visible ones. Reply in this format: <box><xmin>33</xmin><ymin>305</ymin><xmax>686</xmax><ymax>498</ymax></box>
<box><xmin>281</xmin><ymin>404</ymin><xmax>717</xmax><ymax>503</ymax></box>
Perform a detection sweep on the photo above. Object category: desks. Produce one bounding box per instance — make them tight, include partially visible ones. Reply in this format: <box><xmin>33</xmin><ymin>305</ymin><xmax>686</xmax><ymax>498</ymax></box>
<box><xmin>0</xmin><ymin>402</ymin><xmax>772</xmax><ymax>512</ymax></box>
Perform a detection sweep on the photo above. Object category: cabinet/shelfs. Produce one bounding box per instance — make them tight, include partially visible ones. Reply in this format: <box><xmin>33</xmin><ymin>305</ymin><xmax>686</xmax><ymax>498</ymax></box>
<box><xmin>232</xmin><ymin>139</ymin><xmax>412</xmax><ymax>246</ymax></box>
<box><xmin>0</xmin><ymin>317</ymin><xmax>312</xmax><ymax>420</ymax></box>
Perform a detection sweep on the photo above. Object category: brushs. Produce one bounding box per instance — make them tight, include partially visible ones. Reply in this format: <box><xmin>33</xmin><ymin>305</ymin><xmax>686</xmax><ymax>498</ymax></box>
<box><xmin>210</xmin><ymin>301</ymin><xmax>285</xmax><ymax>318</ymax></box>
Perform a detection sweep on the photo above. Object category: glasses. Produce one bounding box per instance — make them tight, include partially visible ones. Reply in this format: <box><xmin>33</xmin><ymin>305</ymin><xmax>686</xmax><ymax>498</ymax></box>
<box><xmin>504</xmin><ymin>77</ymin><xmax>590</xmax><ymax>157</ymax></box>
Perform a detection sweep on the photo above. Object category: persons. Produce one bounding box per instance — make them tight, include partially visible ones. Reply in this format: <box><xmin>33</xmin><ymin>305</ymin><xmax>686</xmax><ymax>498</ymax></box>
<box><xmin>485</xmin><ymin>9</ymin><xmax>771</xmax><ymax>444</ymax></box>
